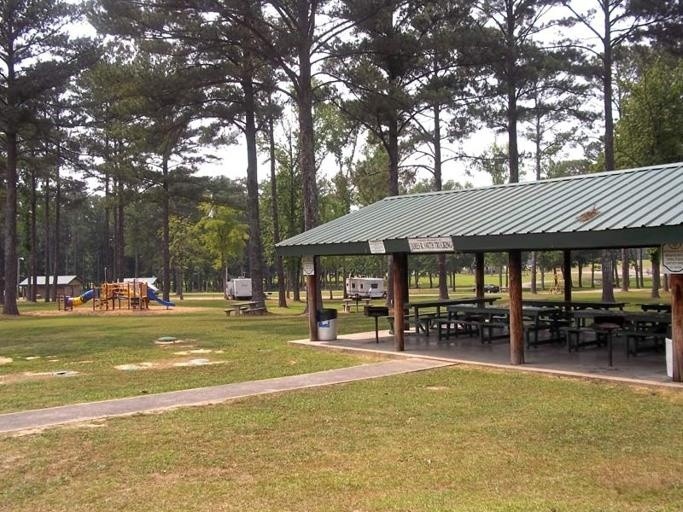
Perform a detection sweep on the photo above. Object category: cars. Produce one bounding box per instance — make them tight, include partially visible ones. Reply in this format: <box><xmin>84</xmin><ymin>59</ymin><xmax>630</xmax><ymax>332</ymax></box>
<box><xmin>472</xmin><ymin>284</ymin><xmax>499</xmax><ymax>294</ymax></box>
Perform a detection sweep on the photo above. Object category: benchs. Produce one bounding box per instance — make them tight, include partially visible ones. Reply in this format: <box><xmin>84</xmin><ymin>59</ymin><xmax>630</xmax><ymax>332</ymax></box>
<box><xmin>242</xmin><ymin>306</ymin><xmax>266</xmax><ymax>317</ymax></box>
<box><xmin>223</xmin><ymin>306</ymin><xmax>249</xmax><ymax>317</ymax></box>
<box><xmin>561</xmin><ymin>324</ymin><xmax>670</xmax><ymax>367</ymax></box>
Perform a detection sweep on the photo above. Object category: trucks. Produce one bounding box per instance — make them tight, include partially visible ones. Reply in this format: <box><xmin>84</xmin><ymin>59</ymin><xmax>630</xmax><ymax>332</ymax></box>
<box><xmin>345</xmin><ymin>277</ymin><xmax>387</xmax><ymax>300</ymax></box>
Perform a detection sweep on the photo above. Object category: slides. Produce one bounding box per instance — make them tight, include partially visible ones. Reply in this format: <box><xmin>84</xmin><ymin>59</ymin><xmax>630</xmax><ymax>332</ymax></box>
<box><xmin>148</xmin><ymin>289</ymin><xmax>175</xmax><ymax>306</ymax></box>
<box><xmin>65</xmin><ymin>287</ymin><xmax>100</xmax><ymax>306</ymax></box>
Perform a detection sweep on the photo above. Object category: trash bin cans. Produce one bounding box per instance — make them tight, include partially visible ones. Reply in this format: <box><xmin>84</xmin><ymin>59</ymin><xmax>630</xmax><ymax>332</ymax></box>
<box><xmin>316</xmin><ymin>309</ymin><xmax>337</xmax><ymax>341</ymax></box>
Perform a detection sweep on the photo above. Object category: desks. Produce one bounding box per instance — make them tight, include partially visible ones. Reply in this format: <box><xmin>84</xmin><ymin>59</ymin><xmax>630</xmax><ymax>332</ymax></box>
<box><xmin>408</xmin><ymin>296</ymin><xmax>630</xmax><ymax>351</ymax></box>
<box><xmin>574</xmin><ymin>303</ymin><xmax>673</xmax><ymax>348</ymax></box>
<box><xmin>231</xmin><ymin>301</ymin><xmax>264</xmax><ymax>317</ymax></box>
